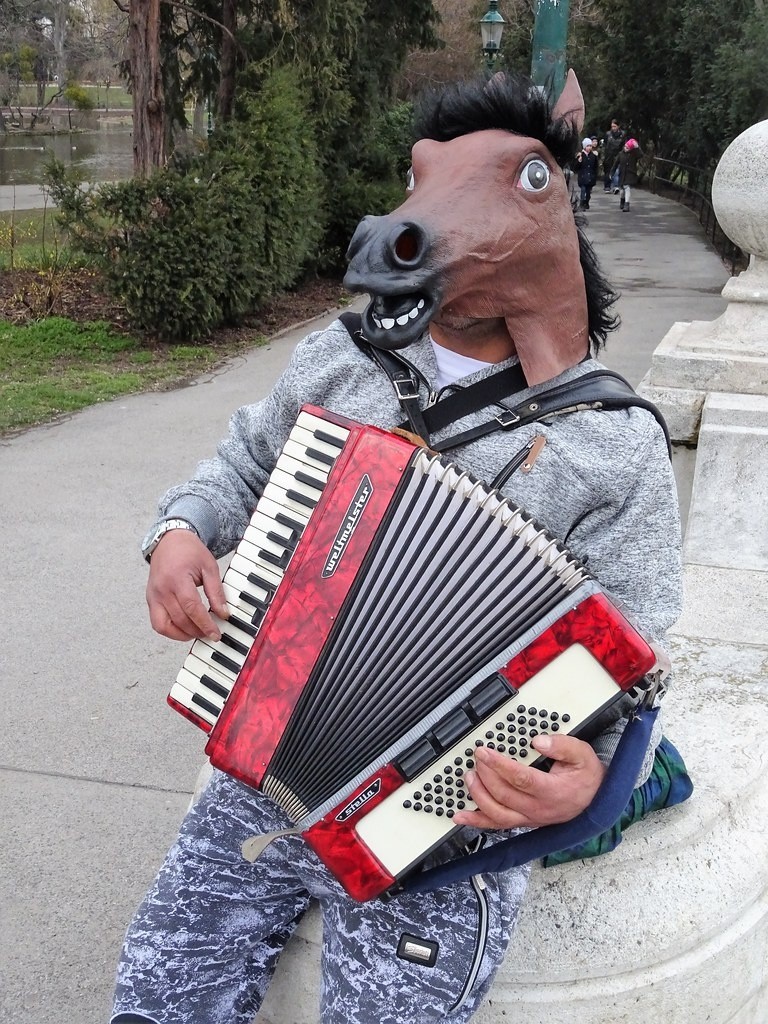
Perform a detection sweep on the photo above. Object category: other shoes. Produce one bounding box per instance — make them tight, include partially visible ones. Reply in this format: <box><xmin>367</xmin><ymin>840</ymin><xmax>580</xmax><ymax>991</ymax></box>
<box><xmin>605</xmin><ymin>189</ymin><xmax>610</xmax><ymax>194</ymax></box>
<box><xmin>613</xmin><ymin>188</ymin><xmax>620</xmax><ymax>194</ymax></box>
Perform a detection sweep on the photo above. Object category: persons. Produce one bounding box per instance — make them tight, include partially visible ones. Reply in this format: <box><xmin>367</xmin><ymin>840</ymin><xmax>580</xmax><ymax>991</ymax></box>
<box><xmin>109</xmin><ymin>69</ymin><xmax>681</xmax><ymax>1024</ymax></box>
<box><xmin>604</xmin><ymin>119</ymin><xmax>625</xmax><ymax>194</ymax></box>
<box><xmin>572</xmin><ymin>138</ymin><xmax>599</xmax><ymax>212</ymax></box>
<box><xmin>590</xmin><ymin>136</ymin><xmax>601</xmax><ymax>160</ymax></box>
<box><xmin>609</xmin><ymin>139</ymin><xmax>644</xmax><ymax>212</ymax></box>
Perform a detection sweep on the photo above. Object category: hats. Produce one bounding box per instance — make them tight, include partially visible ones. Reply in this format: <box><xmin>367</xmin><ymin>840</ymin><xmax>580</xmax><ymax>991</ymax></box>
<box><xmin>627</xmin><ymin>139</ymin><xmax>638</xmax><ymax>149</ymax></box>
<box><xmin>591</xmin><ymin>136</ymin><xmax>598</xmax><ymax>139</ymax></box>
<box><xmin>582</xmin><ymin>138</ymin><xmax>592</xmax><ymax>148</ymax></box>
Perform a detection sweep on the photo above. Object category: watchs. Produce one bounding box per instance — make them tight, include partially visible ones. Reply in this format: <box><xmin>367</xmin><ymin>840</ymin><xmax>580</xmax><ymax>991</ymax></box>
<box><xmin>141</xmin><ymin>519</ymin><xmax>198</xmax><ymax>565</ymax></box>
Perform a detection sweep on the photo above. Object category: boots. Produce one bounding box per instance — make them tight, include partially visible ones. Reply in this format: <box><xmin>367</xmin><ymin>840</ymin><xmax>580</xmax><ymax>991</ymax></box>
<box><xmin>579</xmin><ymin>200</ymin><xmax>586</xmax><ymax>212</ymax></box>
<box><xmin>623</xmin><ymin>202</ymin><xmax>629</xmax><ymax>212</ymax></box>
<box><xmin>586</xmin><ymin>197</ymin><xmax>591</xmax><ymax>209</ymax></box>
<box><xmin>620</xmin><ymin>198</ymin><xmax>625</xmax><ymax>209</ymax></box>
